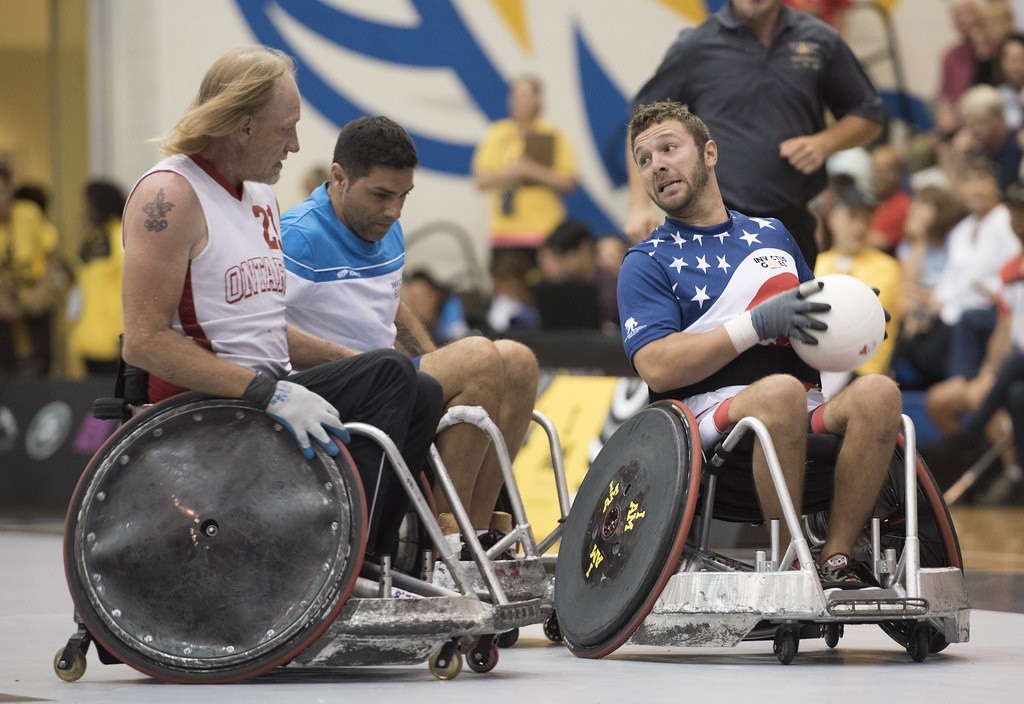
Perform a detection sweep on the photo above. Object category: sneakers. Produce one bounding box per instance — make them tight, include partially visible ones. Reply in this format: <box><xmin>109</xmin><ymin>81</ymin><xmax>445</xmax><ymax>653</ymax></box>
<box><xmin>821</xmin><ymin>552</ymin><xmax>863</xmax><ymax>587</ymax></box>
<box><xmin>791</xmin><ymin>556</ymin><xmax>828</xmax><ymax>587</ymax></box>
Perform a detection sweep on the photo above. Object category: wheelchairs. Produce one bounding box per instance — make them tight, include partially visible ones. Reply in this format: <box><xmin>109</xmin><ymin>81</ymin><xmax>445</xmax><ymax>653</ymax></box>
<box><xmin>553</xmin><ymin>388</ymin><xmax>972</xmax><ymax>666</ymax></box>
<box><xmin>53</xmin><ymin>329</ymin><xmax>569</xmax><ymax>683</ymax></box>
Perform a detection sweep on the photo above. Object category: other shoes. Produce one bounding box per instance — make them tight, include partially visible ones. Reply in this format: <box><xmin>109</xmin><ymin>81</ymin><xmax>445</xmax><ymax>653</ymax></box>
<box><xmin>478</xmin><ymin>529</ymin><xmax>515</xmax><ymax>560</ymax></box>
<box><xmin>436</xmin><ymin>548</ymin><xmax>472</xmax><ymax>561</ymax></box>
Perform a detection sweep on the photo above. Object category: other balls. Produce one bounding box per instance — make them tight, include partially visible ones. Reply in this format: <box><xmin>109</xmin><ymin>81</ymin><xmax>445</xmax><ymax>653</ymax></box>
<box><xmin>789</xmin><ymin>272</ymin><xmax>886</xmax><ymax>372</ymax></box>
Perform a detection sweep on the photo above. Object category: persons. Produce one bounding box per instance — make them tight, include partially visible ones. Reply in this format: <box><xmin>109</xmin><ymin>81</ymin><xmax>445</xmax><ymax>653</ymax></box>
<box><xmin>631</xmin><ymin>0</ymin><xmax>893</xmax><ymax>528</ymax></box>
<box><xmin>1</xmin><ymin>1</ymin><xmax>1024</xmax><ymax>521</ymax></box>
<box><xmin>119</xmin><ymin>44</ymin><xmax>447</xmax><ymax>579</ymax></box>
<box><xmin>611</xmin><ymin>100</ymin><xmax>904</xmax><ymax>590</ymax></box>
<box><xmin>274</xmin><ymin>115</ymin><xmax>539</xmax><ymax>559</ymax></box>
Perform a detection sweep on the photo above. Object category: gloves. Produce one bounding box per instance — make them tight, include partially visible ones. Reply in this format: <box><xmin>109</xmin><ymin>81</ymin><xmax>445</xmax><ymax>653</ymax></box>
<box><xmin>872</xmin><ymin>288</ymin><xmax>891</xmax><ymax>339</ymax></box>
<box><xmin>750</xmin><ymin>280</ymin><xmax>831</xmax><ymax>345</ymax></box>
<box><xmin>264</xmin><ymin>379</ymin><xmax>351</xmax><ymax>460</ymax></box>
